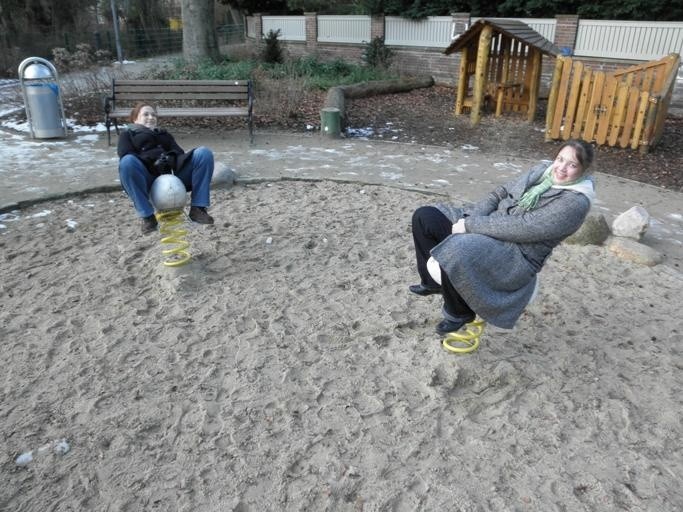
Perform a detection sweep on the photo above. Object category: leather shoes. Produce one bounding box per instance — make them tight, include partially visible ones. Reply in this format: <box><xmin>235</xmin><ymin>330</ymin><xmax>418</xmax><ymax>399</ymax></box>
<box><xmin>409</xmin><ymin>283</ymin><xmax>443</xmax><ymax>296</ymax></box>
<box><xmin>435</xmin><ymin>314</ymin><xmax>476</xmax><ymax>335</ymax></box>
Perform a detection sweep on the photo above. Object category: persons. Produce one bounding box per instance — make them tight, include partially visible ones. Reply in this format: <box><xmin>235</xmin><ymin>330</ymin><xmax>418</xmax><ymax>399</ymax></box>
<box><xmin>408</xmin><ymin>137</ymin><xmax>596</xmax><ymax>334</ymax></box>
<box><xmin>116</xmin><ymin>101</ymin><xmax>214</xmax><ymax>234</ymax></box>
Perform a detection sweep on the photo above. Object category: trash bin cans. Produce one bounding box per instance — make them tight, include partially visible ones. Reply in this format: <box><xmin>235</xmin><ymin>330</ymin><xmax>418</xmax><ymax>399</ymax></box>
<box><xmin>18</xmin><ymin>56</ymin><xmax>69</xmax><ymax>139</ymax></box>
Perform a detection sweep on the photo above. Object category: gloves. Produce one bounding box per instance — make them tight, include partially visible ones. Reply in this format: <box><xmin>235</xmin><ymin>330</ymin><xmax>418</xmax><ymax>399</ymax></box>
<box><xmin>154</xmin><ymin>154</ymin><xmax>176</xmax><ymax>171</ymax></box>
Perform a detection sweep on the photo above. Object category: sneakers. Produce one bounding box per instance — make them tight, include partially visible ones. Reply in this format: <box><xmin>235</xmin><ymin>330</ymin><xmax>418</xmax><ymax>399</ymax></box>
<box><xmin>189</xmin><ymin>206</ymin><xmax>215</xmax><ymax>225</ymax></box>
<box><xmin>141</xmin><ymin>215</ymin><xmax>158</xmax><ymax>234</ymax></box>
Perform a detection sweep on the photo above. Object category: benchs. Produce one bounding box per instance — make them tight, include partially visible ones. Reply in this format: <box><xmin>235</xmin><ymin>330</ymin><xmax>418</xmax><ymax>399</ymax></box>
<box><xmin>100</xmin><ymin>76</ymin><xmax>254</xmax><ymax>139</ymax></box>
